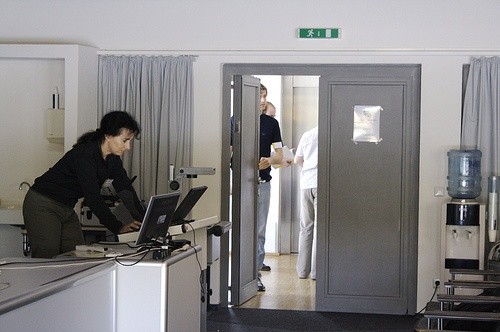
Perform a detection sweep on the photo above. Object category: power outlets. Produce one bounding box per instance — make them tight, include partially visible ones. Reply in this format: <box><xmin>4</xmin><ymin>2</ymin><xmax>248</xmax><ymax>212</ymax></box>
<box><xmin>433</xmin><ymin>279</ymin><xmax>441</xmax><ymax>288</ymax></box>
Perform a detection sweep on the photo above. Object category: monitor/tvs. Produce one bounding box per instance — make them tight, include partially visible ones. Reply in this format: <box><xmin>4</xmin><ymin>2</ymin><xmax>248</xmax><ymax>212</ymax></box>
<box><xmin>171</xmin><ymin>186</ymin><xmax>208</xmax><ymax>225</ymax></box>
<box><xmin>135</xmin><ymin>192</ymin><xmax>180</xmax><ymax>246</ymax></box>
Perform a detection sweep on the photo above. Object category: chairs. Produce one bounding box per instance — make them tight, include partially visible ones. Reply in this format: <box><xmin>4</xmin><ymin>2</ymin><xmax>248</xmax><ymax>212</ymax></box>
<box><xmin>415</xmin><ymin>268</ymin><xmax>500</xmax><ymax>332</ymax></box>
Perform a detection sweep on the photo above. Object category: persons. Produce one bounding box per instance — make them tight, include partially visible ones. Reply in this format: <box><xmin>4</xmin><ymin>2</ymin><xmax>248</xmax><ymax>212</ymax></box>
<box><xmin>230</xmin><ymin>82</ymin><xmax>283</xmax><ymax>291</ymax></box>
<box><xmin>257</xmin><ymin>101</ymin><xmax>293</xmax><ymax>271</ymax></box>
<box><xmin>22</xmin><ymin>110</ymin><xmax>145</xmax><ymax>258</ymax></box>
<box><xmin>294</xmin><ymin>126</ymin><xmax>319</xmax><ymax>280</ymax></box>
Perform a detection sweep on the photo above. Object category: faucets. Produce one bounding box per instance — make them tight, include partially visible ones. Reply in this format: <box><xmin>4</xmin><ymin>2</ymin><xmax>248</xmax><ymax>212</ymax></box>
<box><xmin>18</xmin><ymin>182</ymin><xmax>31</xmax><ymax>191</ymax></box>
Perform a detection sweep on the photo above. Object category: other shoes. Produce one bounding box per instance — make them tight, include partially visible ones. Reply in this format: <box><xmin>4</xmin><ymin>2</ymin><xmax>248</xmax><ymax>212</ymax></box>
<box><xmin>257</xmin><ymin>276</ymin><xmax>266</xmax><ymax>292</ymax></box>
<box><xmin>262</xmin><ymin>263</ymin><xmax>271</xmax><ymax>271</ymax></box>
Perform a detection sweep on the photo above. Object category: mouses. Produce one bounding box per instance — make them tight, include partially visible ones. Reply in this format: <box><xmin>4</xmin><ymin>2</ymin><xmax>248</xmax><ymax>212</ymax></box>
<box><xmin>130</xmin><ymin>223</ymin><xmax>141</xmax><ymax>232</ymax></box>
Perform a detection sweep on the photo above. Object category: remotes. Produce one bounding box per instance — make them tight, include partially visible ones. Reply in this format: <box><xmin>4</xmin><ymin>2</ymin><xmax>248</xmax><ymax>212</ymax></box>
<box><xmin>76</xmin><ymin>245</ymin><xmax>108</xmax><ymax>253</ymax></box>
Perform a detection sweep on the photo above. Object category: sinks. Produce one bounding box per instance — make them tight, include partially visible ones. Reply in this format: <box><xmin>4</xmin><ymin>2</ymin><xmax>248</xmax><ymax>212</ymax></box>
<box><xmin>0</xmin><ymin>204</ymin><xmax>24</xmax><ymax>225</ymax></box>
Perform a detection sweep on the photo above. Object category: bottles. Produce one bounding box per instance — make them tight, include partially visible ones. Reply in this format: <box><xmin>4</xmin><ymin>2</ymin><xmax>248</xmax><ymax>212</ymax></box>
<box><xmin>446</xmin><ymin>149</ymin><xmax>482</xmax><ymax>199</ymax></box>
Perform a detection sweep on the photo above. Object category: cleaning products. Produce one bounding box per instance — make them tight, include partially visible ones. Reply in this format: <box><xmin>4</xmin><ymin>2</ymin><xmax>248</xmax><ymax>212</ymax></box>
<box><xmin>52</xmin><ymin>88</ymin><xmax>59</xmax><ymax>108</ymax></box>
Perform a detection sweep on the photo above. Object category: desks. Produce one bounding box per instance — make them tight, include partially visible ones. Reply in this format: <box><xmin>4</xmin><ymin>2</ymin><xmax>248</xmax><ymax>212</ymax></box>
<box><xmin>0</xmin><ymin>216</ymin><xmax>233</xmax><ymax>332</ymax></box>
